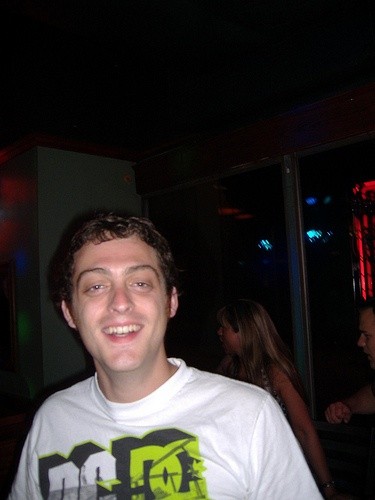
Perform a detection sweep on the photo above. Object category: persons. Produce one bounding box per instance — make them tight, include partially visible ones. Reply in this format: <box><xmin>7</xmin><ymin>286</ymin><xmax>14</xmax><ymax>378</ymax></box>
<box><xmin>6</xmin><ymin>208</ymin><xmax>326</xmax><ymax>500</ymax></box>
<box><xmin>211</xmin><ymin>298</ymin><xmax>354</xmax><ymax>500</ymax></box>
<box><xmin>321</xmin><ymin>297</ymin><xmax>374</xmax><ymax>426</ymax></box>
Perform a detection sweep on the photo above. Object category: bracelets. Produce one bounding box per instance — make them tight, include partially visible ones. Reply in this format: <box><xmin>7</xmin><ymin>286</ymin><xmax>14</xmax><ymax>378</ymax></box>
<box><xmin>320</xmin><ymin>479</ymin><xmax>335</xmax><ymax>489</ymax></box>
